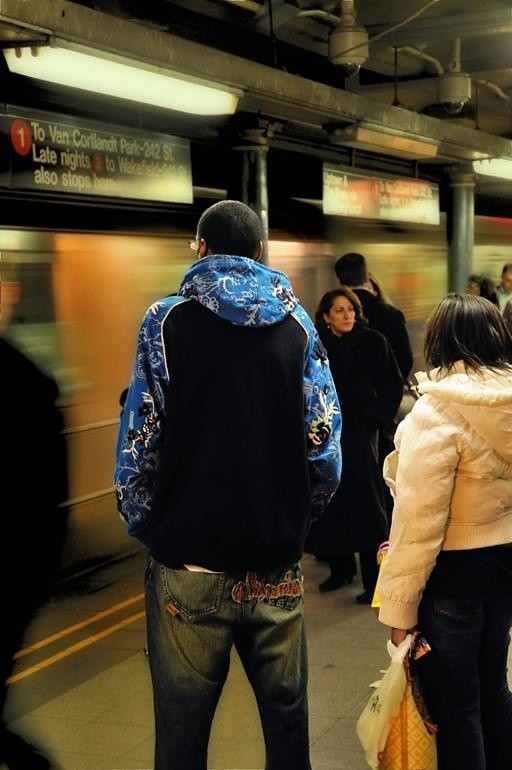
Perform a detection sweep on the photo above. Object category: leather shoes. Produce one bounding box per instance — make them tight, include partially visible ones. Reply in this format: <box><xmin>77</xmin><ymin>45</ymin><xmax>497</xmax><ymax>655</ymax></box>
<box><xmin>319</xmin><ymin>575</ymin><xmax>353</xmax><ymax>592</ymax></box>
<box><xmin>356</xmin><ymin>590</ymin><xmax>375</xmax><ymax>604</ymax></box>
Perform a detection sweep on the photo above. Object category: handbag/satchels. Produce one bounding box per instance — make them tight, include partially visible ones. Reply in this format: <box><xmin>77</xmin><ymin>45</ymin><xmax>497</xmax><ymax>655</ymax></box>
<box><xmin>379</xmin><ymin>668</ymin><xmax>441</xmax><ymax>770</ymax></box>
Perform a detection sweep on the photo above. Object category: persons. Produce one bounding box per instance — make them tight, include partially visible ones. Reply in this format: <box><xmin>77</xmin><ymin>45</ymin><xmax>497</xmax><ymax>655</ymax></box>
<box><xmin>367</xmin><ymin>272</ymin><xmax>394</xmax><ymax>305</ymax></box>
<box><xmin>493</xmin><ymin>260</ymin><xmax>512</xmax><ymax>363</ymax></box>
<box><xmin>460</xmin><ymin>273</ymin><xmax>496</xmax><ymax>301</ymax></box>
<box><xmin>315</xmin><ymin>291</ymin><xmax>404</xmax><ymax>605</ymax></box>
<box><xmin>377</xmin><ymin>292</ymin><xmax>512</xmax><ymax>770</ymax></box>
<box><xmin>113</xmin><ymin>199</ymin><xmax>345</xmax><ymax>770</ymax></box>
<box><xmin>333</xmin><ymin>249</ymin><xmax>415</xmax><ymax>377</ymax></box>
<box><xmin>0</xmin><ymin>334</ymin><xmax>74</xmax><ymax>769</ymax></box>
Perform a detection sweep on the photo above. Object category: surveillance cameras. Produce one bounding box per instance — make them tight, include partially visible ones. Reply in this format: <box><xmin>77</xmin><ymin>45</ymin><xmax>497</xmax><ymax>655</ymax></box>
<box><xmin>436</xmin><ymin>72</ymin><xmax>472</xmax><ymax>115</ymax></box>
<box><xmin>328</xmin><ymin>27</ymin><xmax>370</xmax><ymax>78</ymax></box>
